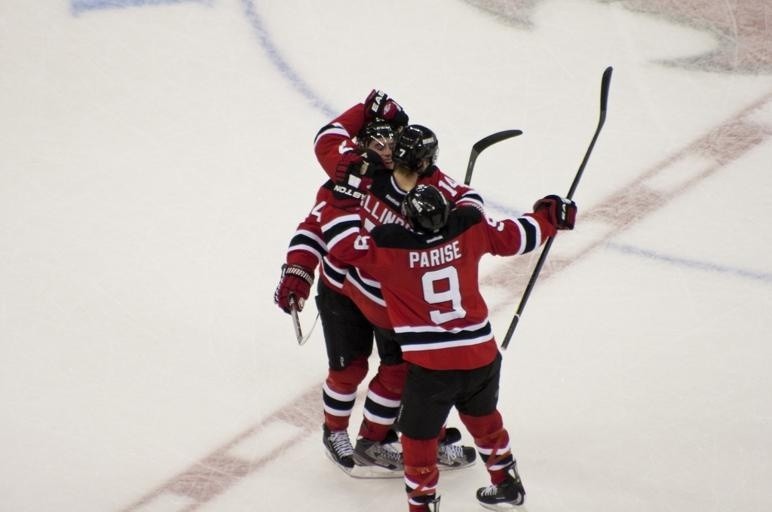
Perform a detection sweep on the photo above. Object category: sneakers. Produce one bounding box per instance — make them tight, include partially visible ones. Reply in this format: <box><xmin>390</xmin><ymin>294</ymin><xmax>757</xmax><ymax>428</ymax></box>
<box><xmin>323</xmin><ymin>429</ymin><xmax>522</xmax><ymax>504</ymax></box>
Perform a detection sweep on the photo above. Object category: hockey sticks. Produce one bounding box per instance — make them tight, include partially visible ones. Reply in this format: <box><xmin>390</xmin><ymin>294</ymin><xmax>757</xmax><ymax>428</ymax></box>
<box><xmin>464</xmin><ymin>131</ymin><xmax>522</xmax><ymax>185</ymax></box>
<box><xmin>288</xmin><ymin>291</ymin><xmax>320</xmax><ymax>347</ymax></box>
<box><xmin>501</xmin><ymin>67</ymin><xmax>612</xmax><ymax>351</ymax></box>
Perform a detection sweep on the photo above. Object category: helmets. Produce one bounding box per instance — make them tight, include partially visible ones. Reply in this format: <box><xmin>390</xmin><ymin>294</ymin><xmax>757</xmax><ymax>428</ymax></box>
<box><xmin>391</xmin><ymin>124</ymin><xmax>438</xmax><ymax>171</ymax></box>
<box><xmin>358</xmin><ymin>119</ymin><xmax>397</xmax><ymax>148</ymax></box>
<box><xmin>405</xmin><ymin>184</ymin><xmax>449</xmax><ymax>232</ymax></box>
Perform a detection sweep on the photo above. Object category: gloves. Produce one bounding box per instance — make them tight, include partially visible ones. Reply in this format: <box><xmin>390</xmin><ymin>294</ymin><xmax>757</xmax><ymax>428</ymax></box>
<box><xmin>274</xmin><ymin>265</ymin><xmax>314</xmax><ymax>314</ymax></box>
<box><xmin>365</xmin><ymin>90</ymin><xmax>408</xmax><ymax>130</ymax></box>
<box><xmin>334</xmin><ymin>150</ymin><xmax>375</xmax><ymax>197</ymax></box>
<box><xmin>533</xmin><ymin>195</ymin><xmax>577</xmax><ymax>229</ymax></box>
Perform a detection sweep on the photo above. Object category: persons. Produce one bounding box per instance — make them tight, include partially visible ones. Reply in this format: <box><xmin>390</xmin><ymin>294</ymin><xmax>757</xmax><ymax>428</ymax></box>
<box><xmin>272</xmin><ymin>115</ymin><xmax>462</xmax><ymax>470</ymax></box>
<box><xmin>312</xmin><ymin>87</ymin><xmax>486</xmax><ymax>473</ymax></box>
<box><xmin>316</xmin><ymin>149</ymin><xmax>578</xmax><ymax>512</ymax></box>
